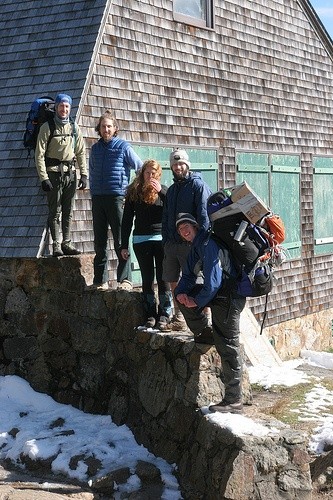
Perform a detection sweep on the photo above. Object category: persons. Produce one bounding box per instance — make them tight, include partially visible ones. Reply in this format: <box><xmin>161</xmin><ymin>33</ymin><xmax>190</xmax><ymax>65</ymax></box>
<box><xmin>89</xmin><ymin>109</ymin><xmax>135</xmax><ymax>292</ymax></box>
<box><xmin>161</xmin><ymin>145</ymin><xmax>209</xmax><ymax>331</ymax></box>
<box><xmin>35</xmin><ymin>94</ymin><xmax>88</xmax><ymax>258</ymax></box>
<box><xmin>174</xmin><ymin>211</ymin><xmax>246</xmax><ymax>412</ymax></box>
<box><xmin>120</xmin><ymin>161</ymin><xmax>171</xmax><ymax>331</ymax></box>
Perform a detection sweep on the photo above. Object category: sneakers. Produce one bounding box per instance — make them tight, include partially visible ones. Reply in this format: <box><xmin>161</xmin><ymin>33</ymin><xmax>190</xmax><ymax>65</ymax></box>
<box><xmin>209</xmin><ymin>399</ymin><xmax>242</xmax><ymax>413</ymax></box>
<box><xmin>194</xmin><ymin>325</ymin><xmax>216</xmax><ymax>344</ymax></box>
<box><xmin>61</xmin><ymin>239</ymin><xmax>81</xmax><ymax>255</ymax></box>
<box><xmin>53</xmin><ymin>241</ymin><xmax>64</xmax><ymax>256</ymax></box>
<box><xmin>159</xmin><ymin>314</ymin><xmax>188</xmax><ymax>332</ymax></box>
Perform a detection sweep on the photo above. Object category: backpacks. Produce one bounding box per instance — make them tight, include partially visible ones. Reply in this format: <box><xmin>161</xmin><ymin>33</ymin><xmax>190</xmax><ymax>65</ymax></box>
<box><xmin>203</xmin><ymin>181</ymin><xmax>286</xmax><ymax>297</ymax></box>
<box><xmin>23</xmin><ymin>96</ymin><xmax>77</xmax><ymax>167</ymax></box>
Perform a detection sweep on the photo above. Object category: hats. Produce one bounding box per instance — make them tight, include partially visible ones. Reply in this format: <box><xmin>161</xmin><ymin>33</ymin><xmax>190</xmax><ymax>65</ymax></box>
<box><xmin>176</xmin><ymin>213</ymin><xmax>198</xmax><ymax>229</ymax></box>
<box><xmin>170</xmin><ymin>150</ymin><xmax>190</xmax><ymax>170</ymax></box>
<box><xmin>55</xmin><ymin>94</ymin><xmax>73</xmax><ymax>106</ymax></box>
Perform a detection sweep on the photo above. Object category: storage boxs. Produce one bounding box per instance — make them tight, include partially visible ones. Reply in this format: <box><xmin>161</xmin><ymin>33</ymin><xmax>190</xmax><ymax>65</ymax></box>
<box><xmin>231</xmin><ymin>180</ymin><xmax>269</xmax><ymax>225</ymax></box>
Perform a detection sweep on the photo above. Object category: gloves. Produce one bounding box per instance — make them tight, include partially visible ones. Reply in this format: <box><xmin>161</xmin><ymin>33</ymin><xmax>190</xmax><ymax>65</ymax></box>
<box><xmin>41</xmin><ymin>179</ymin><xmax>53</xmax><ymax>192</ymax></box>
<box><xmin>78</xmin><ymin>175</ymin><xmax>87</xmax><ymax>190</ymax></box>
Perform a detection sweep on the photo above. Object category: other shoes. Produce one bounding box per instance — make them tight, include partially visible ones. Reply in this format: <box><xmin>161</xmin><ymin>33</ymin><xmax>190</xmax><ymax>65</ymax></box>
<box><xmin>117</xmin><ymin>280</ymin><xmax>133</xmax><ymax>291</ymax></box>
<box><xmin>146</xmin><ymin>314</ymin><xmax>156</xmax><ymax>327</ymax></box>
<box><xmin>84</xmin><ymin>282</ymin><xmax>108</xmax><ymax>291</ymax></box>
<box><xmin>157</xmin><ymin>316</ymin><xmax>168</xmax><ymax>326</ymax></box>
